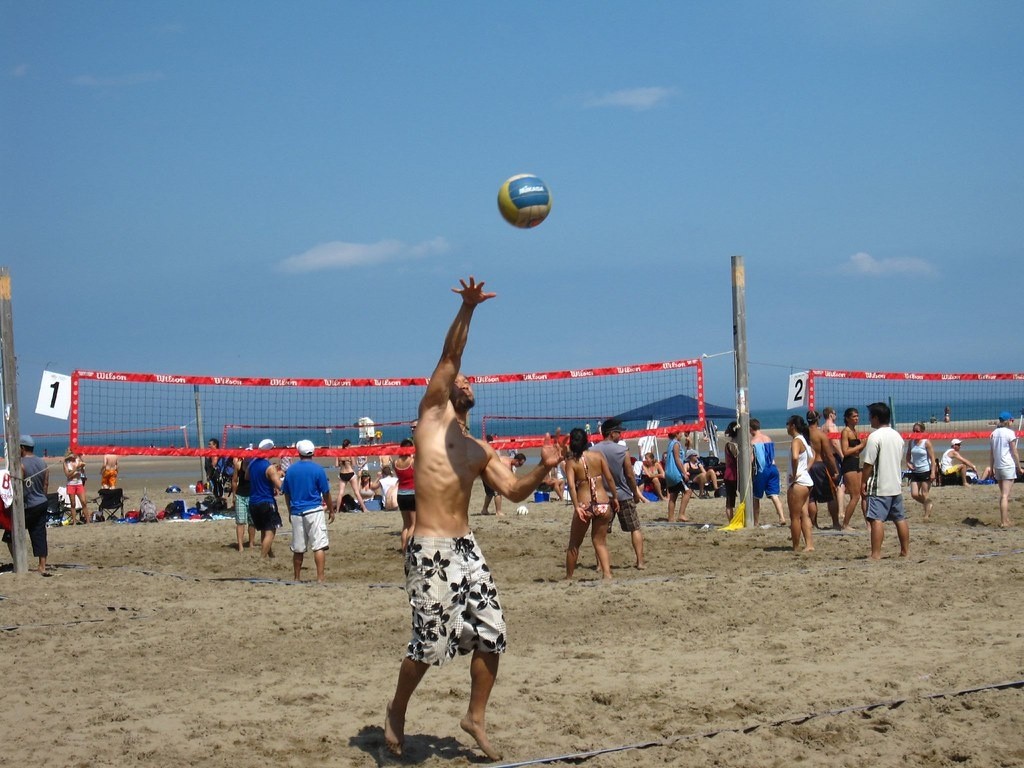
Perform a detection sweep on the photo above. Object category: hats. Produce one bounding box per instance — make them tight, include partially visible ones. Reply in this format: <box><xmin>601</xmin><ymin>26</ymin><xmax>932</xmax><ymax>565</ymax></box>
<box><xmin>19</xmin><ymin>434</ymin><xmax>35</xmax><ymax>446</ymax></box>
<box><xmin>950</xmin><ymin>438</ymin><xmax>962</xmax><ymax>446</ymax></box>
<box><xmin>296</xmin><ymin>439</ymin><xmax>315</xmax><ymax>456</ymax></box>
<box><xmin>601</xmin><ymin>417</ymin><xmax>627</xmax><ymax>432</ymax></box>
<box><xmin>806</xmin><ymin>410</ymin><xmax>820</xmax><ymax>421</ymax></box>
<box><xmin>258</xmin><ymin>439</ymin><xmax>274</xmax><ymax>450</ymax></box>
<box><xmin>999</xmin><ymin>411</ymin><xmax>1015</xmax><ymax>422</ymax></box>
<box><xmin>686</xmin><ymin>449</ymin><xmax>697</xmax><ymax>459</ymax></box>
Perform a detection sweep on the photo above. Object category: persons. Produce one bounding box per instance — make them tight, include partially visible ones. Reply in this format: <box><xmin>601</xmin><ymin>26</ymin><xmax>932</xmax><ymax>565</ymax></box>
<box><xmin>282</xmin><ymin>438</ymin><xmax>335</xmax><ymax>584</ymax></box>
<box><xmin>786</xmin><ymin>403</ymin><xmax>934</xmax><ymax>558</ymax></box>
<box><xmin>336</xmin><ymin>420</ymin><xmax>626</xmax><ymax>553</ymax></box>
<box><xmin>588</xmin><ymin>419</ymin><xmax>648</xmax><ymax>572</ymax></box>
<box><xmin>99</xmin><ymin>444</ymin><xmax>119</xmax><ymax>490</ymax></box>
<box><xmin>387</xmin><ymin>275</ymin><xmax>570</xmax><ymax>761</ymax></box>
<box><xmin>981</xmin><ymin>411</ymin><xmax>1024</xmax><ymax>527</ymax></box>
<box><xmin>205</xmin><ymin>438</ymin><xmax>293</xmax><ymax>559</ymax></box>
<box><xmin>63</xmin><ymin>452</ymin><xmax>90</xmax><ymax>527</ymax></box>
<box><xmin>2</xmin><ymin>435</ymin><xmax>49</xmax><ymax>575</ymax></box>
<box><xmin>634</xmin><ymin>419</ymin><xmax>785</xmax><ymax>529</ymax></box>
<box><xmin>561</xmin><ymin>427</ymin><xmax>620</xmax><ymax>580</ymax></box>
<box><xmin>940</xmin><ymin>438</ymin><xmax>978</xmax><ymax>487</ymax></box>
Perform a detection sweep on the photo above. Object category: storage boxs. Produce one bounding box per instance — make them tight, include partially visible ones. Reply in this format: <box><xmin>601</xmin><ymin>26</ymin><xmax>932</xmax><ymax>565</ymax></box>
<box><xmin>535</xmin><ymin>492</ymin><xmax>549</xmax><ymax>501</ymax></box>
<box><xmin>364</xmin><ymin>499</ymin><xmax>382</xmax><ymax>511</ymax></box>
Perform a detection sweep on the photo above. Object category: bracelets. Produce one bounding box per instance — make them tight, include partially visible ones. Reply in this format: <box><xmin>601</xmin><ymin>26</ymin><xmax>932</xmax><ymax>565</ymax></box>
<box><xmin>972</xmin><ymin>466</ymin><xmax>976</xmax><ymax>469</ymax></box>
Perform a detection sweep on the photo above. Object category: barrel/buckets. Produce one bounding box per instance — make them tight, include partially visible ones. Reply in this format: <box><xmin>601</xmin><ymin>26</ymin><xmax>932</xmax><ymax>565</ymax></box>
<box><xmin>534</xmin><ymin>492</ymin><xmax>549</xmax><ymax>503</ymax></box>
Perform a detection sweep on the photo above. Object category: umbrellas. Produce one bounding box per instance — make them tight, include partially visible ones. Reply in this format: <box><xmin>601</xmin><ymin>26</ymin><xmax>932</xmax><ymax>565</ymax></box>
<box><xmin>613</xmin><ymin>394</ymin><xmax>751</xmax><ymax>442</ymax></box>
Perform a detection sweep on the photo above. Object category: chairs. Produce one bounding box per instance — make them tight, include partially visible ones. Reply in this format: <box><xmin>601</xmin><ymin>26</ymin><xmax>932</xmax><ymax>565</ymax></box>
<box><xmin>936</xmin><ymin>458</ymin><xmax>970</xmax><ymax>486</ymax></box>
<box><xmin>46</xmin><ymin>486</ymin><xmax>85</xmax><ymax>526</ymax></box>
<box><xmin>555</xmin><ymin>464</ymin><xmax>572</xmax><ymax>505</ymax></box>
<box><xmin>684</xmin><ymin>460</ymin><xmax>710</xmax><ymax>498</ymax></box>
<box><xmin>91</xmin><ymin>488</ymin><xmax>130</xmax><ymax>521</ymax></box>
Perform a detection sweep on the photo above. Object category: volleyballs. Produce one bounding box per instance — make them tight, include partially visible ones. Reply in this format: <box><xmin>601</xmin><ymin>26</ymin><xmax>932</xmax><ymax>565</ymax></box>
<box><xmin>496</xmin><ymin>172</ymin><xmax>553</xmax><ymax>230</ymax></box>
<box><xmin>516</xmin><ymin>506</ymin><xmax>528</xmax><ymax>515</ymax></box>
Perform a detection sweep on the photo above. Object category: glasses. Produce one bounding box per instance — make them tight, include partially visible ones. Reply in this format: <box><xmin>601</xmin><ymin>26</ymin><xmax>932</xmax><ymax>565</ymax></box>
<box><xmin>692</xmin><ymin>455</ymin><xmax>697</xmax><ymax>457</ymax></box>
<box><xmin>786</xmin><ymin>422</ymin><xmax>789</xmax><ymax>425</ymax></box>
<box><xmin>519</xmin><ymin>461</ymin><xmax>523</xmax><ymax>466</ymax></box>
<box><xmin>832</xmin><ymin>413</ymin><xmax>836</xmax><ymax>416</ymax></box>
<box><xmin>1010</xmin><ymin>418</ymin><xmax>1015</xmax><ymax>422</ymax></box>
